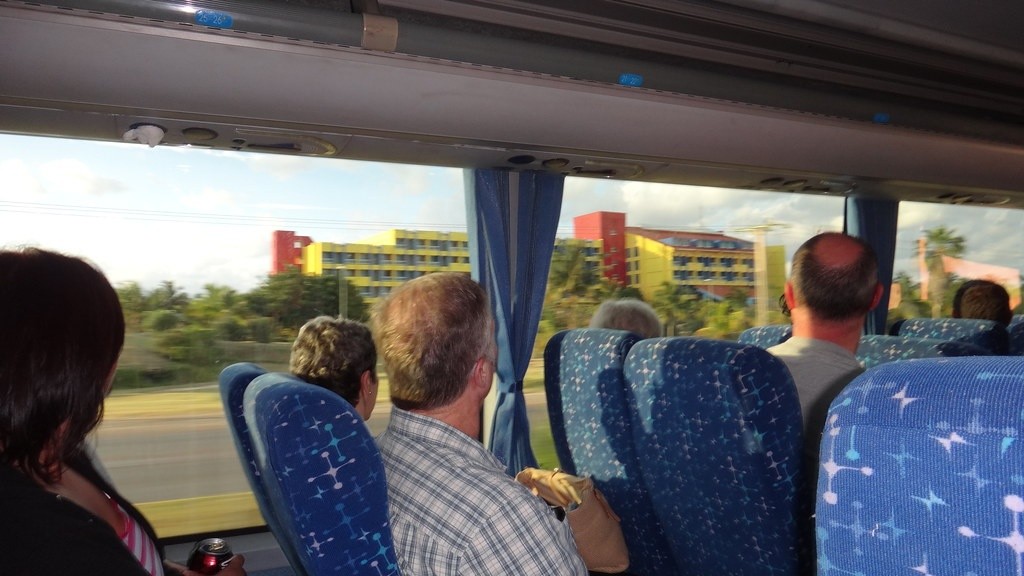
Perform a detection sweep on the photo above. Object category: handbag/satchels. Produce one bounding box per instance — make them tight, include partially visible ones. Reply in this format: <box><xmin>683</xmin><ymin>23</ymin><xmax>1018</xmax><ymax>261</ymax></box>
<box><xmin>512</xmin><ymin>466</ymin><xmax>630</xmax><ymax>573</ymax></box>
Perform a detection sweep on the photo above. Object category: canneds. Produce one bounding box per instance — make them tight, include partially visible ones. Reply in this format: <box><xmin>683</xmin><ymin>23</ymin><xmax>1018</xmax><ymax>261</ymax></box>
<box><xmin>189</xmin><ymin>537</ymin><xmax>233</xmax><ymax>576</ymax></box>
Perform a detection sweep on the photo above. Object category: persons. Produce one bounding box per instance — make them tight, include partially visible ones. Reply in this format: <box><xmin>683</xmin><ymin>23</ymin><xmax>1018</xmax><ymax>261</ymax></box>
<box><xmin>0</xmin><ymin>246</ymin><xmax>252</xmax><ymax>576</ymax></box>
<box><xmin>766</xmin><ymin>232</ymin><xmax>885</xmax><ymax>470</ymax></box>
<box><xmin>953</xmin><ymin>279</ymin><xmax>1013</xmax><ymax>328</ymax></box>
<box><xmin>590</xmin><ymin>299</ymin><xmax>664</xmax><ymax>339</ymax></box>
<box><xmin>371</xmin><ymin>274</ymin><xmax>588</xmax><ymax>575</ymax></box>
<box><xmin>288</xmin><ymin>315</ymin><xmax>378</xmax><ymax>423</ymax></box>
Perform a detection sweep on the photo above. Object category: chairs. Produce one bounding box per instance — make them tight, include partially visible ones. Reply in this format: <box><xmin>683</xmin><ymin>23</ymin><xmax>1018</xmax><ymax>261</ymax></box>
<box><xmin>543</xmin><ymin>329</ymin><xmax>676</xmax><ymax>576</ymax></box>
<box><xmin>815</xmin><ymin>356</ymin><xmax>1024</xmax><ymax>576</ymax></box>
<box><xmin>854</xmin><ymin>335</ymin><xmax>995</xmax><ymax>372</ymax></box>
<box><xmin>889</xmin><ymin>318</ymin><xmax>1011</xmax><ymax>356</ymax></box>
<box><xmin>1006</xmin><ymin>313</ymin><xmax>1024</xmax><ymax>356</ymax></box>
<box><xmin>242</xmin><ymin>373</ymin><xmax>401</xmax><ymax>576</ymax></box>
<box><xmin>624</xmin><ymin>337</ymin><xmax>815</xmax><ymax>576</ymax></box>
<box><xmin>737</xmin><ymin>325</ymin><xmax>793</xmax><ymax>351</ymax></box>
<box><xmin>217</xmin><ymin>362</ymin><xmax>326</xmax><ymax>576</ymax></box>
